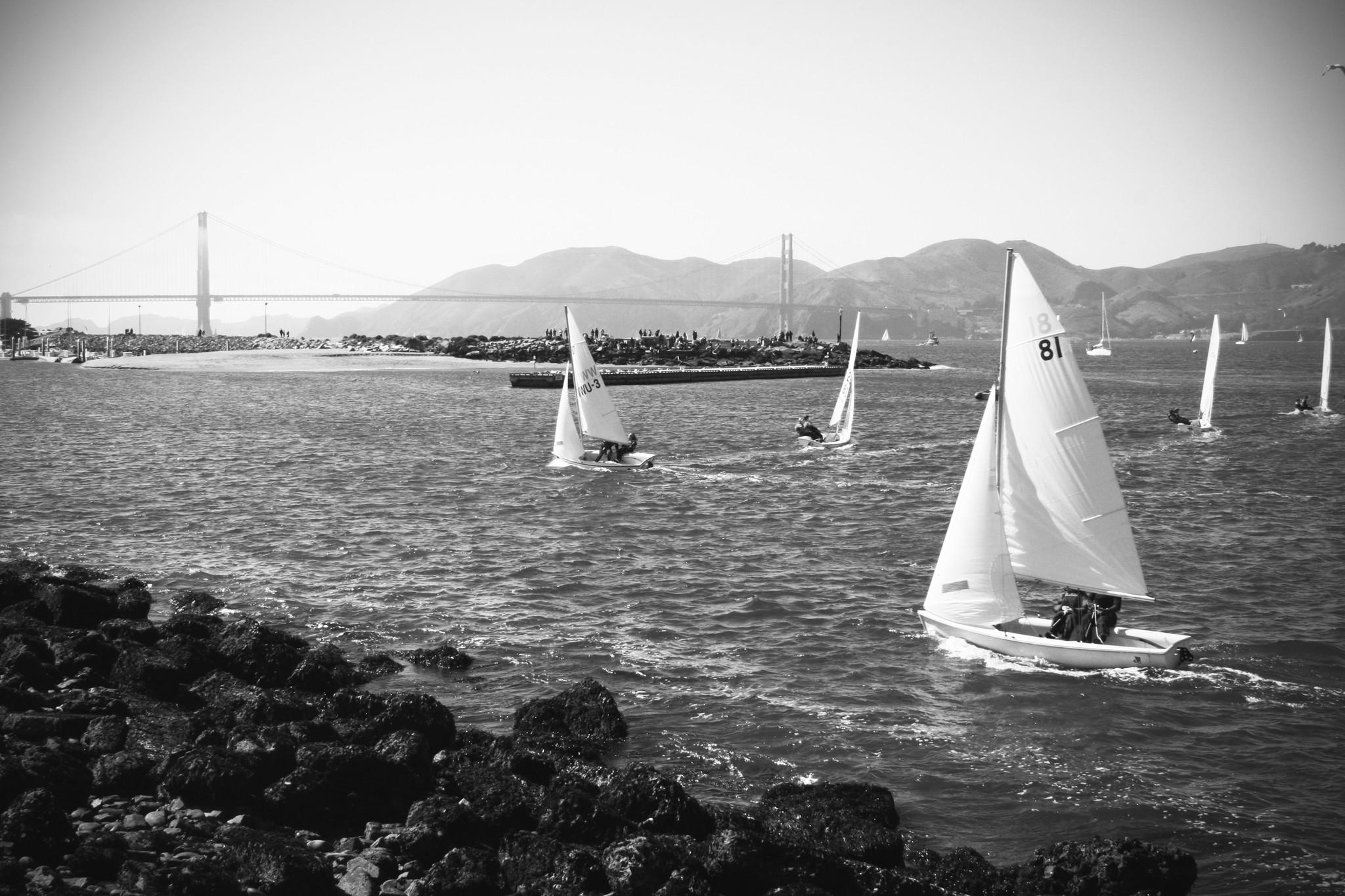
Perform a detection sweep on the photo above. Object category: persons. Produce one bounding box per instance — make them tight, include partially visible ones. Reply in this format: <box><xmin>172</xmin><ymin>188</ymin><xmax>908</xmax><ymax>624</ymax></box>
<box><xmin>198</xmin><ymin>329</ymin><xmax>205</xmax><ymax>337</ymax></box>
<box><xmin>564</xmin><ymin>328</ymin><xmax>568</xmax><ymax>339</ymax></box>
<box><xmin>591</xmin><ymin>328</ymin><xmax>713</xmax><ymax>354</ymax></box>
<box><xmin>795</xmin><ymin>415</ymin><xmax>826</xmax><ymax>443</ymax></box>
<box><xmin>1295</xmin><ymin>398</ymin><xmax>1305</xmax><ymax>411</ymax></box>
<box><xmin>757</xmin><ymin>330</ymin><xmax>793</xmax><ymax>347</ymax></box>
<box><xmin>1080</xmin><ymin>593</ymin><xmax>1121</xmax><ymax>644</ymax></box>
<box><xmin>546</xmin><ymin>329</ymin><xmax>563</xmax><ymax>337</ymax></box>
<box><xmin>837</xmin><ymin>334</ymin><xmax>840</xmax><ymax>342</ymax></box>
<box><xmin>1168</xmin><ymin>408</ymin><xmax>1191</xmax><ymax>425</ymax></box>
<box><xmin>614</xmin><ymin>433</ymin><xmax>637</xmax><ymax>463</ymax></box>
<box><xmin>731</xmin><ymin>338</ymin><xmax>750</xmax><ymax>348</ymax></box>
<box><xmin>1301</xmin><ymin>396</ymin><xmax>1309</xmax><ymax>408</ymax></box>
<box><xmin>280</xmin><ymin>329</ymin><xmax>289</xmax><ymax>337</ymax></box>
<box><xmin>595</xmin><ymin>440</ymin><xmax>619</xmax><ymax>462</ymax></box>
<box><xmin>126</xmin><ymin>328</ymin><xmax>133</xmax><ymax>334</ymax></box>
<box><xmin>1045</xmin><ymin>587</ymin><xmax>1088</xmax><ymax>641</ymax></box>
<box><xmin>798</xmin><ymin>330</ymin><xmax>818</xmax><ymax>344</ymax></box>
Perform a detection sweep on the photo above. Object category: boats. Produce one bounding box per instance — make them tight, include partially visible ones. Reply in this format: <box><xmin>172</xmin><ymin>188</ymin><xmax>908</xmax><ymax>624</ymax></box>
<box><xmin>925</xmin><ymin>332</ymin><xmax>939</xmax><ymax>345</ymax></box>
<box><xmin>38</xmin><ymin>355</ymin><xmax>60</xmax><ymax>362</ymax></box>
<box><xmin>59</xmin><ymin>356</ymin><xmax>85</xmax><ymax>364</ymax></box>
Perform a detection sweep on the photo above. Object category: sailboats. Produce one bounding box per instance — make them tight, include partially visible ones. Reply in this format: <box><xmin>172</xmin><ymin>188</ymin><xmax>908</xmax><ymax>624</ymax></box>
<box><xmin>549</xmin><ymin>304</ymin><xmax>658</xmax><ymax>470</ymax></box>
<box><xmin>1297</xmin><ymin>334</ymin><xmax>1303</xmax><ymax>343</ymax></box>
<box><xmin>1299</xmin><ymin>314</ymin><xmax>1334</xmax><ymax>416</ymax></box>
<box><xmin>1235</xmin><ymin>322</ymin><xmax>1248</xmax><ymax>345</ymax></box>
<box><xmin>878</xmin><ymin>328</ymin><xmax>890</xmax><ymax>342</ymax></box>
<box><xmin>796</xmin><ymin>310</ymin><xmax>863</xmax><ymax>447</ymax></box>
<box><xmin>1174</xmin><ymin>312</ymin><xmax>1224</xmax><ymax>435</ymax></box>
<box><xmin>914</xmin><ymin>247</ymin><xmax>1198</xmax><ymax>671</ymax></box>
<box><xmin>1086</xmin><ymin>291</ymin><xmax>1112</xmax><ymax>357</ymax></box>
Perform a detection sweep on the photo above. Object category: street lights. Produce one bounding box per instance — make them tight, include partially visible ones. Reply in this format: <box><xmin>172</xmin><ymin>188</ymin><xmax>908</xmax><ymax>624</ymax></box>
<box><xmin>138</xmin><ymin>305</ymin><xmax>141</xmax><ymax>334</ymax></box>
<box><xmin>264</xmin><ymin>302</ymin><xmax>268</xmax><ymax>335</ymax></box>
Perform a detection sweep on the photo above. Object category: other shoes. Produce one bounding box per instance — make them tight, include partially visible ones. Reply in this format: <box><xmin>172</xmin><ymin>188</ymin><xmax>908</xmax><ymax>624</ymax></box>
<box><xmin>1062</xmin><ymin>637</ymin><xmax>1069</xmax><ymax>641</ymax></box>
<box><xmin>1091</xmin><ymin>640</ymin><xmax>1100</xmax><ymax>644</ymax></box>
<box><xmin>1078</xmin><ymin>638</ymin><xmax>1087</xmax><ymax>642</ymax></box>
<box><xmin>1045</xmin><ymin>632</ymin><xmax>1058</xmax><ymax>639</ymax></box>
